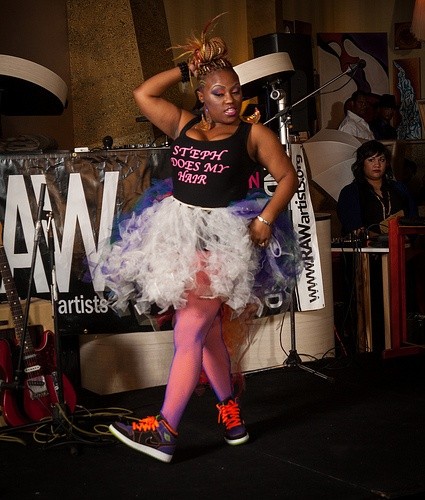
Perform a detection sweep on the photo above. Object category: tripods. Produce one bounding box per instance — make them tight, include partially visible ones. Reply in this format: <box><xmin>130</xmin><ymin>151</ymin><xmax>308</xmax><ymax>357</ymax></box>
<box><xmin>234</xmin><ymin>61</ymin><xmax>360</xmax><ymax>384</ymax></box>
<box><xmin>0</xmin><ymin>183</ymin><xmax>125</xmax><ymax>457</ymax></box>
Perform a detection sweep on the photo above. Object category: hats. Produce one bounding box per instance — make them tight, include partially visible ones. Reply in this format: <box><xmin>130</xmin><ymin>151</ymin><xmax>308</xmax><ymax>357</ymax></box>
<box><xmin>371</xmin><ymin>94</ymin><xmax>401</xmax><ymax>109</ymax></box>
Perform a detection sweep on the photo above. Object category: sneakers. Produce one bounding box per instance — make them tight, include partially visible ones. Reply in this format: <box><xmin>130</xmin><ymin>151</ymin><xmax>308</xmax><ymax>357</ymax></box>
<box><xmin>216</xmin><ymin>396</ymin><xmax>249</xmax><ymax>445</ymax></box>
<box><xmin>108</xmin><ymin>410</ymin><xmax>179</xmax><ymax>463</ymax></box>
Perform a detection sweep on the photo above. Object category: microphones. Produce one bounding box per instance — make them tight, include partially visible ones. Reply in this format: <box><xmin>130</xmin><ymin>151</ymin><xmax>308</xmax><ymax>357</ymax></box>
<box><xmin>0</xmin><ymin>379</ymin><xmax>25</xmax><ymax>389</ymax></box>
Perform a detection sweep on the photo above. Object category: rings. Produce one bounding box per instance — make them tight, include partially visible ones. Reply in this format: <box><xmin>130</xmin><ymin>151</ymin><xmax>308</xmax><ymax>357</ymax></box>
<box><xmin>259</xmin><ymin>242</ymin><xmax>264</xmax><ymax>247</ymax></box>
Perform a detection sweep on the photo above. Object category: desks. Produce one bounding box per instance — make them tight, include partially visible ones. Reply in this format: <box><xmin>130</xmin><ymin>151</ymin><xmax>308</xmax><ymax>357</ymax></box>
<box><xmin>331</xmin><ymin>242</ymin><xmax>411</xmax><ymax>353</ymax></box>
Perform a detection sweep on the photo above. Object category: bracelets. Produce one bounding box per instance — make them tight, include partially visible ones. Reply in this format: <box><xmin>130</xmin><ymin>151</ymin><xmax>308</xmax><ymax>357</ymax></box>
<box><xmin>178</xmin><ymin>63</ymin><xmax>190</xmax><ymax>83</ymax></box>
<box><xmin>257</xmin><ymin>215</ymin><xmax>274</xmax><ymax>228</ymax></box>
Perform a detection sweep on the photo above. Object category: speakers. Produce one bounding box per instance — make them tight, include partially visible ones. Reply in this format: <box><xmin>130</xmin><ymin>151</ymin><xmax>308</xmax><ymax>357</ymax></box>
<box><xmin>252</xmin><ymin>30</ymin><xmax>311</xmax><ymax>139</ymax></box>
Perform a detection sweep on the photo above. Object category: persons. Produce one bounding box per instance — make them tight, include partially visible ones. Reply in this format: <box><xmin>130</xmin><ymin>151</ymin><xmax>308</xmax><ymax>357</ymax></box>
<box><xmin>336</xmin><ymin>140</ymin><xmax>420</xmax><ymax>337</ymax></box>
<box><xmin>367</xmin><ymin>94</ymin><xmax>399</xmax><ymax>140</ymax></box>
<box><xmin>77</xmin><ymin>11</ymin><xmax>304</xmax><ymax>463</ymax></box>
<box><xmin>337</xmin><ymin>90</ymin><xmax>375</xmax><ymax>140</ymax></box>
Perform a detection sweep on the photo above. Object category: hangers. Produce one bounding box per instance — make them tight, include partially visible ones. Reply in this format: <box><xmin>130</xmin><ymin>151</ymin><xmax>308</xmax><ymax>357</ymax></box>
<box><xmin>0</xmin><ymin>151</ymin><xmax>142</xmax><ymax>181</ymax></box>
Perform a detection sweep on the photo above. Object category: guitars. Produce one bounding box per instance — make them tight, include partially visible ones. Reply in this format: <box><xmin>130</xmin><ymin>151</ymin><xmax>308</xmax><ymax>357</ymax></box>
<box><xmin>1</xmin><ymin>246</ymin><xmax>76</xmax><ymax>430</ymax></box>
